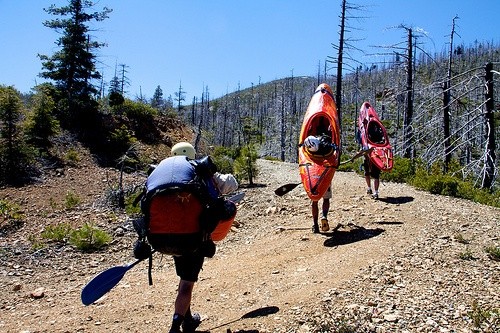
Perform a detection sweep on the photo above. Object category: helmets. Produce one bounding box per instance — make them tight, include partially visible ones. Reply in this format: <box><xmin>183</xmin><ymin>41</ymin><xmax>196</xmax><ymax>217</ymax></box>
<box><xmin>304</xmin><ymin>136</ymin><xmax>319</xmax><ymax>152</ymax></box>
<box><xmin>171</xmin><ymin>142</ymin><xmax>196</xmax><ymax>159</ymax></box>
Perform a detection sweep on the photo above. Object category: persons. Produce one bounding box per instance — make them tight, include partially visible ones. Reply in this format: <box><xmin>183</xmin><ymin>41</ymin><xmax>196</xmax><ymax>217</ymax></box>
<box><xmin>140</xmin><ymin>141</ymin><xmax>239</xmax><ymax>333</ymax></box>
<box><xmin>356</xmin><ymin>102</ymin><xmax>394</xmax><ymax>199</ymax></box>
<box><xmin>299</xmin><ymin>133</ymin><xmax>342</xmax><ymax>235</ymax></box>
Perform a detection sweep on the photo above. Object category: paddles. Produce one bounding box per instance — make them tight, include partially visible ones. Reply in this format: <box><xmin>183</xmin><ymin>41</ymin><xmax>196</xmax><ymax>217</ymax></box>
<box><xmin>81</xmin><ymin>192</ymin><xmax>246</xmax><ymax>306</ymax></box>
<box><xmin>274</xmin><ymin>145</ymin><xmax>376</xmax><ymax>196</ymax></box>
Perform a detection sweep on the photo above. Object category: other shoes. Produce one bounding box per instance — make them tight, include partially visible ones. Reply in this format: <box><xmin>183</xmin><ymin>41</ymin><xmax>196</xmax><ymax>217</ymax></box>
<box><xmin>312</xmin><ymin>225</ymin><xmax>319</xmax><ymax>233</ymax></box>
<box><xmin>182</xmin><ymin>313</ymin><xmax>200</xmax><ymax>330</ymax></box>
<box><xmin>321</xmin><ymin>216</ymin><xmax>329</xmax><ymax>231</ymax></box>
<box><xmin>367</xmin><ymin>190</ymin><xmax>372</xmax><ymax>194</ymax></box>
<box><xmin>372</xmin><ymin>194</ymin><xmax>378</xmax><ymax>199</ymax></box>
